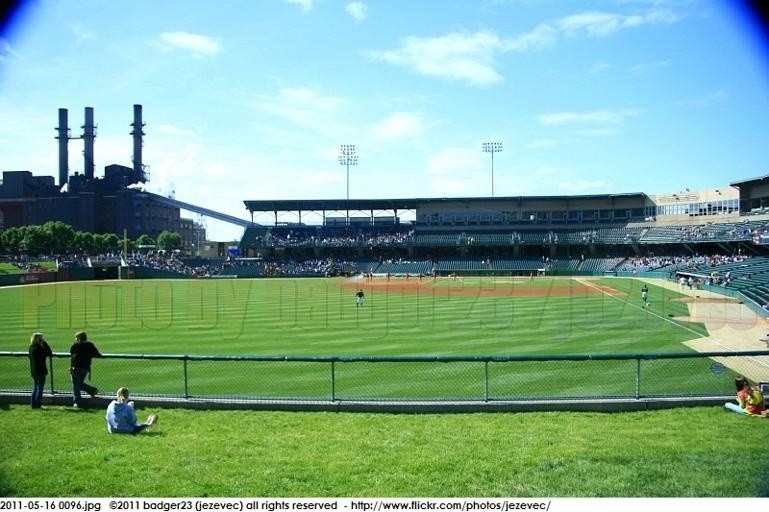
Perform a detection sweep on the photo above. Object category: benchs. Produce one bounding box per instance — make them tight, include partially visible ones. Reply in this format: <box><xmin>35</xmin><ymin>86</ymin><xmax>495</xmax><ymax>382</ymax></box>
<box><xmin>705</xmin><ymin>283</ymin><xmax>769</xmax><ymax>323</ymax></box>
<box><xmin>55</xmin><ymin>219</ymin><xmax>769</xmax><ymax>283</ymax></box>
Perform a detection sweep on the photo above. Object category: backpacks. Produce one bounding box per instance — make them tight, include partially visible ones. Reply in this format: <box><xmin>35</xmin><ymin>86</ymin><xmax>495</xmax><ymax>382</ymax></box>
<box><xmin>744</xmin><ymin>386</ymin><xmax>764</xmax><ymax>407</ymax></box>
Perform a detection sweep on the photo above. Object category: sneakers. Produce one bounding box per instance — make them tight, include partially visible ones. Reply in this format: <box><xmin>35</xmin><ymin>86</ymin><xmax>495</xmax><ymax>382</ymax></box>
<box><xmin>145</xmin><ymin>414</ymin><xmax>159</xmax><ymax>427</ymax></box>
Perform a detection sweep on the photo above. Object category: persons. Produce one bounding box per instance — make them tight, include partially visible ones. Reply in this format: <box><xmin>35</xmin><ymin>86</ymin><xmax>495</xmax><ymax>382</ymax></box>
<box><xmin>723</xmin><ymin>375</ymin><xmax>765</xmax><ymax>416</ymax></box>
<box><xmin>431</xmin><ymin>267</ymin><xmax>438</xmax><ymax>282</ymax></box>
<box><xmin>640</xmin><ymin>285</ymin><xmax>649</xmax><ymax>307</ymax></box>
<box><xmin>28</xmin><ymin>331</ymin><xmax>53</xmax><ymax>411</ymax></box>
<box><xmin>227</xmin><ymin>227</ymin><xmax>420</xmax><ymax>281</ymax></box>
<box><xmin>7</xmin><ymin>247</ymin><xmax>227</xmax><ymax>276</ymax></box>
<box><xmin>106</xmin><ymin>386</ymin><xmax>158</xmax><ymax>434</ymax></box>
<box><xmin>354</xmin><ymin>289</ymin><xmax>366</xmax><ymax>308</ymax></box>
<box><xmin>644</xmin><ymin>223</ymin><xmax>769</xmax><ymax>290</ymax></box>
<box><xmin>451</xmin><ymin>271</ymin><xmax>458</xmax><ymax>284</ymax></box>
<box><xmin>527</xmin><ymin>269</ymin><xmax>535</xmax><ymax>285</ymax></box>
<box><xmin>469</xmin><ymin>229</ymin><xmax>643</xmax><ymax>273</ymax></box>
<box><xmin>68</xmin><ymin>330</ymin><xmax>102</xmax><ymax>409</ymax></box>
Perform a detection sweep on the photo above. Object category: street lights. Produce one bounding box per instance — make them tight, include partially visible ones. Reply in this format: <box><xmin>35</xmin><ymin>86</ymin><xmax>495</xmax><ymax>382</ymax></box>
<box><xmin>481</xmin><ymin>142</ymin><xmax>504</xmax><ymax>197</ymax></box>
<box><xmin>337</xmin><ymin>143</ymin><xmax>359</xmax><ymax>229</ymax></box>
